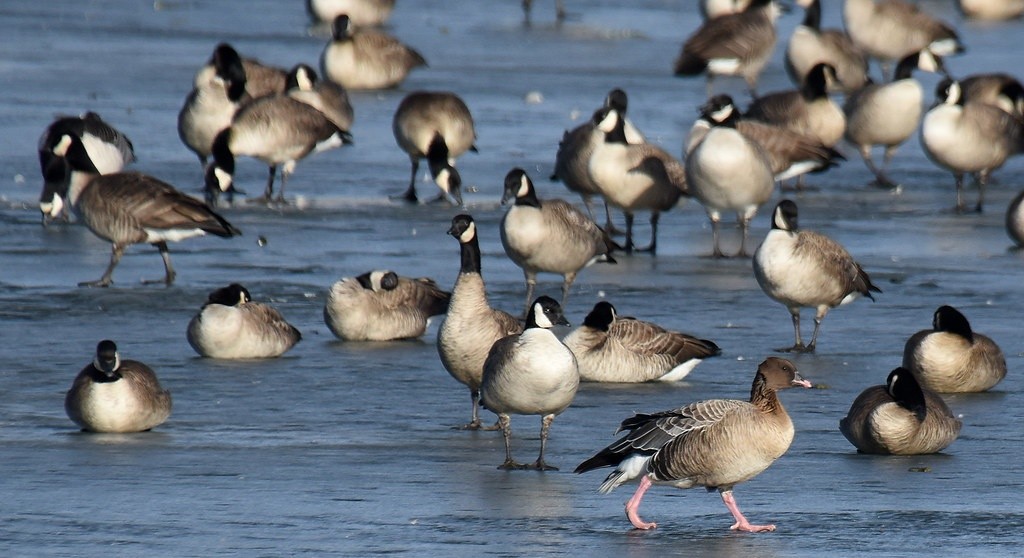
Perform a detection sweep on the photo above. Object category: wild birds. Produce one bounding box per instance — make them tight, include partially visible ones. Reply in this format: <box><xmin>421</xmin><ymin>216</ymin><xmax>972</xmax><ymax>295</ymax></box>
<box><xmin>36</xmin><ymin>0</ymin><xmax>1022</xmax><ymax>534</ymax></box>
